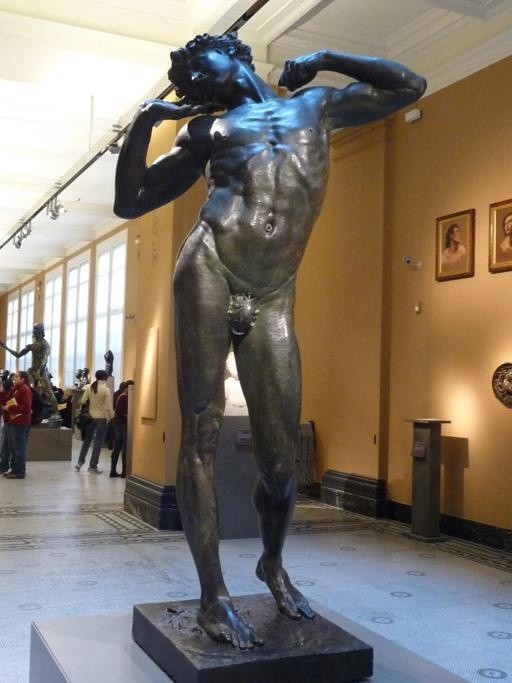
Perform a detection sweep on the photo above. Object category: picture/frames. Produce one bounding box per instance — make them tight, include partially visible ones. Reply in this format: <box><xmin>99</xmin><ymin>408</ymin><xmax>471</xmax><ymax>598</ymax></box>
<box><xmin>488</xmin><ymin>199</ymin><xmax>512</xmax><ymax>273</ymax></box>
<box><xmin>434</xmin><ymin>208</ymin><xmax>475</xmax><ymax>282</ymax></box>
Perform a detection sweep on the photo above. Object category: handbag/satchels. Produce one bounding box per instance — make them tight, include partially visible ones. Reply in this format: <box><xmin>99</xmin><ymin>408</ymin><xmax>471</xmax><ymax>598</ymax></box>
<box><xmin>75</xmin><ymin>398</ymin><xmax>92</xmax><ymax>430</ymax></box>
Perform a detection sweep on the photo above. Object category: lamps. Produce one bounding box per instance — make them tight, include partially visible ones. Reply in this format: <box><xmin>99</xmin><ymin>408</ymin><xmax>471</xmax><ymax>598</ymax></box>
<box><xmin>45</xmin><ymin>194</ymin><xmax>66</xmax><ymax>220</ymax></box>
<box><xmin>12</xmin><ymin>220</ymin><xmax>32</xmax><ymax>249</ymax></box>
<box><xmin>108</xmin><ymin>142</ymin><xmax>121</xmax><ymax>155</ymax></box>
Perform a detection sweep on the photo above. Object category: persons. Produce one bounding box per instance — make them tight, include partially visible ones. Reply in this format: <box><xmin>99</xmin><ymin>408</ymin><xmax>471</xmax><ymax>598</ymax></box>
<box><xmin>113</xmin><ymin>28</ymin><xmax>432</xmax><ymax>654</ymax></box>
<box><xmin>439</xmin><ymin>223</ymin><xmax>466</xmax><ymax>262</ymax></box>
<box><xmin>0</xmin><ymin>320</ymin><xmax>63</xmax><ymax>421</ymax></box>
<box><xmin>0</xmin><ymin>351</ymin><xmax>137</xmax><ymax>480</ymax></box>
<box><xmin>498</xmin><ymin>212</ymin><xmax>512</xmax><ymax>256</ymax></box>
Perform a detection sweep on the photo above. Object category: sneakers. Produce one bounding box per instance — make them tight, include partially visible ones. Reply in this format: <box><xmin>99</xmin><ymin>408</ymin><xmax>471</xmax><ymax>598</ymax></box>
<box><xmin>74</xmin><ymin>463</ymin><xmax>103</xmax><ymax>474</ymax></box>
<box><xmin>0</xmin><ymin>462</ymin><xmax>25</xmax><ymax>479</ymax></box>
<box><xmin>109</xmin><ymin>471</ymin><xmax>126</xmax><ymax>478</ymax></box>
